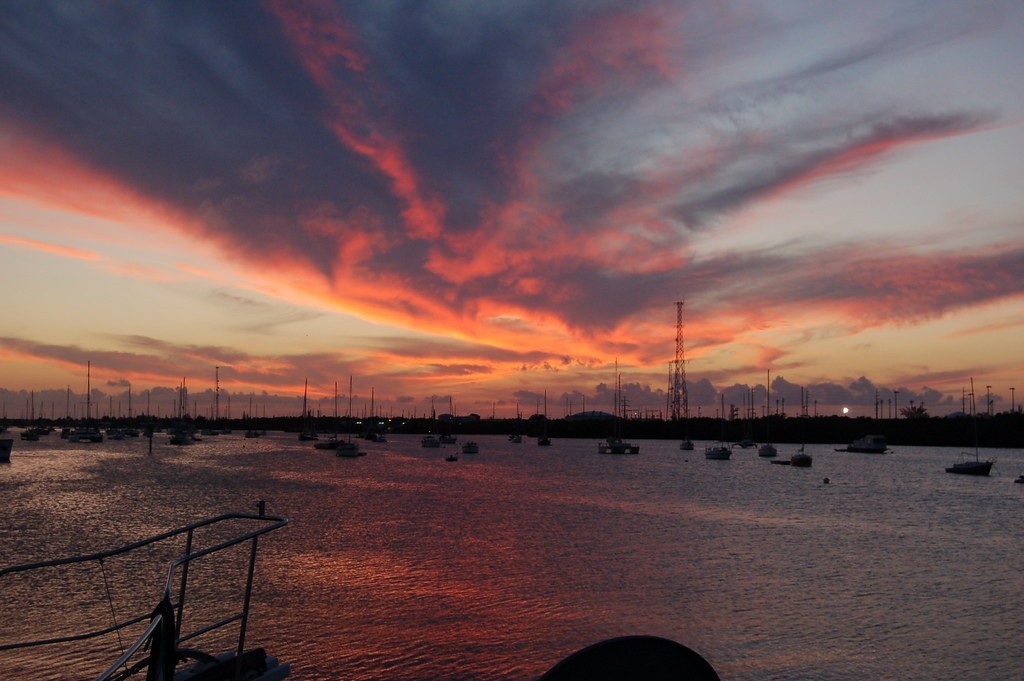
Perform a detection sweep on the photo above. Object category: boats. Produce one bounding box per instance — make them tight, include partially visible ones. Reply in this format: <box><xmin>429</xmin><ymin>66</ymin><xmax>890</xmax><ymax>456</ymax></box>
<box><xmin>704</xmin><ymin>432</ymin><xmax>733</xmax><ymax>459</ymax></box>
<box><xmin>769</xmin><ymin>459</ymin><xmax>791</xmax><ymax>465</ymax></box>
<box><xmin>507</xmin><ymin>430</ymin><xmax>523</xmax><ymax>443</ymax></box>
<box><xmin>462</xmin><ymin>444</ymin><xmax>479</xmax><ymax>453</ymax></box>
<box><xmin>790</xmin><ymin>443</ymin><xmax>813</xmax><ymax>468</ymax></box>
<box><xmin>737</xmin><ymin>438</ymin><xmax>757</xmax><ymax>448</ymax></box>
<box><xmin>439</xmin><ymin>434</ymin><xmax>458</xmax><ymax>445</ymax></box>
<box><xmin>758</xmin><ymin>441</ymin><xmax>776</xmax><ymax>458</ymax></box>
<box><xmin>679</xmin><ymin>438</ymin><xmax>696</xmax><ymax>450</ymax></box>
<box><xmin>421</xmin><ymin>436</ymin><xmax>440</xmax><ymax>448</ymax></box>
<box><xmin>446</xmin><ymin>456</ymin><xmax>457</xmax><ymax>461</ymax></box>
<box><xmin>845</xmin><ymin>434</ymin><xmax>887</xmax><ymax>453</ymax></box>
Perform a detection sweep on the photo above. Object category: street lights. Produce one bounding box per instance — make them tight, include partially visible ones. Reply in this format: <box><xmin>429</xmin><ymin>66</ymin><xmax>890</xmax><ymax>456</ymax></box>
<box><xmin>893</xmin><ymin>390</ymin><xmax>899</xmax><ymax>420</ymax></box>
<box><xmin>967</xmin><ymin>392</ymin><xmax>974</xmax><ymax>419</ymax></box>
<box><xmin>1009</xmin><ymin>387</ymin><xmax>1016</xmax><ymax>412</ymax></box>
<box><xmin>986</xmin><ymin>385</ymin><xmax>993</xmax><ymax>416</ymax></box>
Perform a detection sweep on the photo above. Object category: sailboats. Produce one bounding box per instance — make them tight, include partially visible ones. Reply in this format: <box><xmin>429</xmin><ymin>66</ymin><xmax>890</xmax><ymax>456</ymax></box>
<box><xmin>944</xmin><ymin>377</ymin><xmax>997</xmax><ymax>476</ymax></box>
<box><xmin>299</xmin><ymin>374</ymin><xmax>388</xmax><ymax>457</ymax></box>
<box><xmin>599</xmin><ymin>393</ymin><xmax>640</xmax><ymax>454</ymax></box>
<box><xmin>538</xmin><ymin>390</ymin><xmax>552</xmax><ymax>446</ymax></box>
<box><xmin>0</xmin><ymin>363</ymin><xmax>269</xmax><ymax>446</ymax></box>
